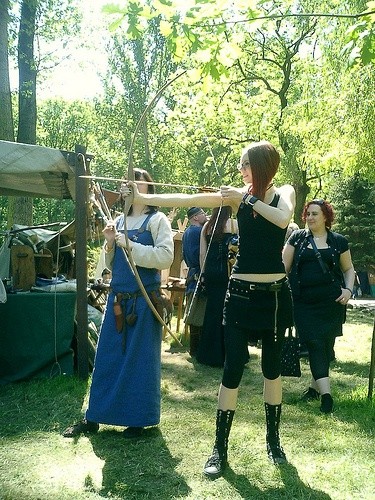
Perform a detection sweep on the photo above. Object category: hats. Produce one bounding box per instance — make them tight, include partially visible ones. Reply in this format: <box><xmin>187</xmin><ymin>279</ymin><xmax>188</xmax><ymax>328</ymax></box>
<box><xmin>187</xmin><ymin>207</ymin><xmax>202</xmax><ymax>218</ymax></box>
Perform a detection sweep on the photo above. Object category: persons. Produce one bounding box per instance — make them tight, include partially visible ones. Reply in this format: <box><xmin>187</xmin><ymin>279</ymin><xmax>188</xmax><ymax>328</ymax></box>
<box><xmin>282</xmin><ymin>198</ymin><xmax>355</xmax><ymax>414</ymax></box>
<box><xmin>182</xmin><ymin>206</ymin><xmax>208</xmax><ymax>355</ymax></box>
<box><xmin>196</xmin><ymin>205</ymin><xmax>239</xmax><ymax>367</ymax></box>
<box><xmin>119</xmin><ymin>141</ymin><xmax>297</xmax><ymax>475</ymax></box>
<box><xmin>63</xmin><ymin>168</ymin><xmax>174</xmax><ymax>439</ymax></box>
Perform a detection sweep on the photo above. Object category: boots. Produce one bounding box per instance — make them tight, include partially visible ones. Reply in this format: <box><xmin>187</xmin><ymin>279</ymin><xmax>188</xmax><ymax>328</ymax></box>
<box><xmin>204</xmin><ymin>408</ymin><xmax>234</xmax><ymax>475</ymax></box>
<box><xmin>264</xmin><ymin>401</ymin><xmax>288</xmax><ymax>467</ymax></box>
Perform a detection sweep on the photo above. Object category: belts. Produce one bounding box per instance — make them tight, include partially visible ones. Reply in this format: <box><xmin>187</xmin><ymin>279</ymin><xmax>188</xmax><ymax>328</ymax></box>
<box><xmin>231</xmin><ymin>280</ymin><xmax>291</xmax><ymax>293</ymax></box>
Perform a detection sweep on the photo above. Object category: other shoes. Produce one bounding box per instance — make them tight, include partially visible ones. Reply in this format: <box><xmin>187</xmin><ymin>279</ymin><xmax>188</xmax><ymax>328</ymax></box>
<box><xmin>300</xmin><ymin>387</ymin><xmax>319</xmax><ymax>401</ymax></box>
<box><xmin>125</xmin><ymin>427</ymin><xmax>142</xmax><ymax>437</ymax></box>
<box><xmin>62</xmin><ymin>420</ymin><xmax>99</xmax><ymax>437</ymax></box>
<box><xmin>320</xmin><ymin>395</ymin><xmax>332</xmax><ymax>414</ymax></box>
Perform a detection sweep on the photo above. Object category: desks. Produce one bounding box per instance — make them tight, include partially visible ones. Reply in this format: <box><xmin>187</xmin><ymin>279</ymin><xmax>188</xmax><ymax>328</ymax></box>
<box><xmin>0</xmin><ymin>291</ymin><xmax>77</xmax><ymax>385</ymax></box>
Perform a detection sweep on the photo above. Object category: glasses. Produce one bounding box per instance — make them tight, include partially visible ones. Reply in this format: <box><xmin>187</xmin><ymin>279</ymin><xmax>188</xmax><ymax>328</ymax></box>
<box><xmin>312</xmin><ymin>199</ymin><xmax>326</xmax><ymax>208</ymax></box>
<box><xmin>237</xmin><ymin>161</ymin><xmax>250</xmax><ymax>171</ymax></box>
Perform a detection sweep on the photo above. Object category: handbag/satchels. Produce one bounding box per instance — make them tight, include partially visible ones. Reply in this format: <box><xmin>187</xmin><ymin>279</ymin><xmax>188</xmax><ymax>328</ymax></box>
<box><xmin>185</xmin><ymin>293</ymin><xmax>209</xmax><ymax>328</ymax></box>
<box><xmin>281</xmin><ymin>326</ymin><xmax>301</xmax><ymax>378</ymax></box>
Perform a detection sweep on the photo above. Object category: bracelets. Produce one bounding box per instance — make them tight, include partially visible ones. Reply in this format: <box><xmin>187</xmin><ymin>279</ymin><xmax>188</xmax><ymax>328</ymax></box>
<box><xmin>247</xmin><ymin>196</ymin><xmax>261</xmax><ymax>209</ymax></box>
<box><xmin>240</xmin><ymin>193</ymin><xmax>249</xmax><ymax>209</ymax></box>
<box><xmin>344</xmin><ymin>288</ymin><xmax>352</xmax><ymax>294</ymax></box>
